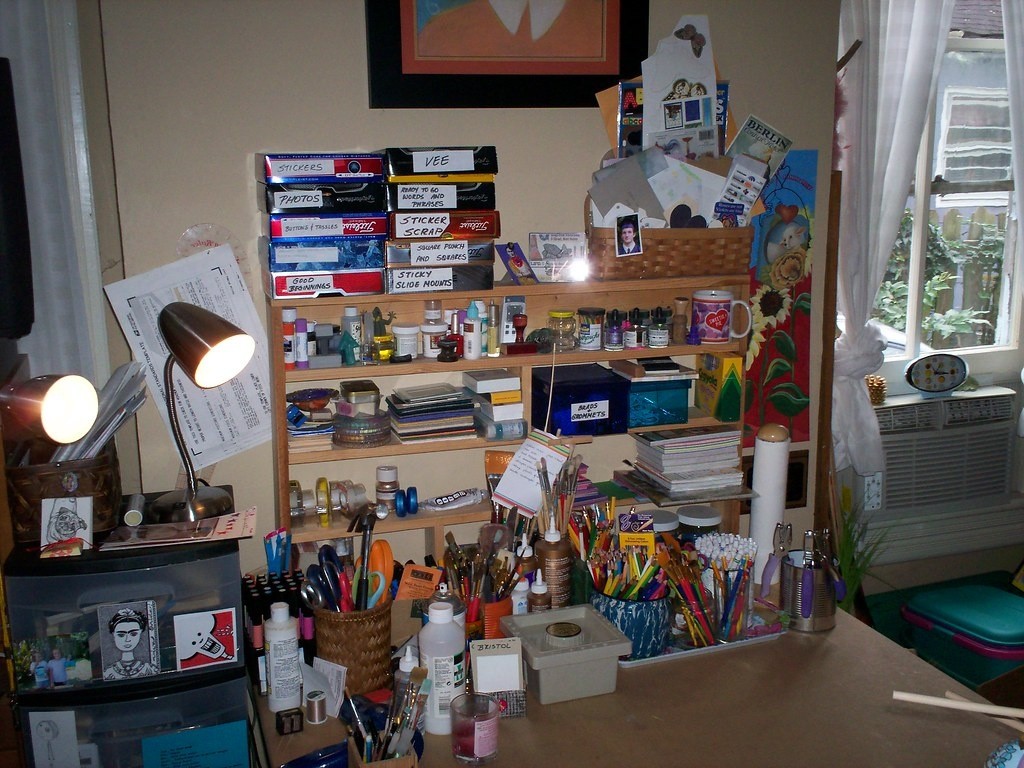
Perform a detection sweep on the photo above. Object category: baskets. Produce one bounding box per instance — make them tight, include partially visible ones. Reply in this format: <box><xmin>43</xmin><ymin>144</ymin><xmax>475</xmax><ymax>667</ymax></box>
<box><xmin>7</xmin><ymin>436</ymin><xmax>122</xmax><ymax>542</ymax></box>
<box><xmin>313</xmin><ymin>591</ymin><xmax>391</xmax><ymax>696</ymax></box>
<box><xmin>584</xmin><ymin>193</ymin><xmax>754</xmax><ymax>281</ymax></box>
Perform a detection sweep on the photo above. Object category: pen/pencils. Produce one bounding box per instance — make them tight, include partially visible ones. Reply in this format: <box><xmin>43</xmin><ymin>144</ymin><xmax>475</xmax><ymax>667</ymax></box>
<box><xmin>695</xmin><ymin>532</ymin><xmax>758</xmax><ymax>575</ymax></box>
<box><xmin>354</xmin><ymin>525</ymin><xmax>372</xmax><ymax>612</ymax></box>
<box><xmin>567</xmin><ymin>497</ymin><xmax>616</xmax><ymax>564</ymax></box>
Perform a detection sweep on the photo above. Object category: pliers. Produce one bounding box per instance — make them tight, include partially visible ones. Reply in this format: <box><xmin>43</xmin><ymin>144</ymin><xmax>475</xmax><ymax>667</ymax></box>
<box><xmin>801</xmin><ymin>527</ymin><xmax>814</xmax><ymax>621</ymax></box>
<box><xmin>815</xmin><ymin>527</ymin><xmax>848</xmax><ymax>605</ymax></box>
<box><xmin>761</xmin><ymin>524</ymin><xmax>794</xmax><ymax>600</ymax></box>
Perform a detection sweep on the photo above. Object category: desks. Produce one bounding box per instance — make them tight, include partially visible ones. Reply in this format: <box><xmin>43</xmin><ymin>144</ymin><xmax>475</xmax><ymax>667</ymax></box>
<box><xmin>248</xmin><ymin>581</ymin><xmax>1023</xmax><ymax>768</ymax></box>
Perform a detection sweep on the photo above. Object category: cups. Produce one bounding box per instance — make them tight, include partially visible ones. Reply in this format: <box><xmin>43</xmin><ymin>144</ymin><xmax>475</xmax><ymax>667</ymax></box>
<box><xmin>593</xmin><ymin>583</ymin><xmax>670</xmax><ymax>659</ymax></box>
<box><xmin>782</xmin><ymin>550</ymin><xmax>838</xmax><ymax>633</ymax></box>
<box><xmin>713</xmin><ymin>569</ymin><xmax>750</xmax><ymax>643</ymax></box>
<box><xmin>693</xmin><ymin>291</ymin><xmax>752</xmax><ymax>344</ymax></box>
<box><xmin>463</xmin><ymin>618</ymin><xmax>482</xmax><ymax>678</ymax></box>
<box><xmin>451</xmin><ymin>694</ymin><xmax>500</xmax><ymax>765</ymax></box>
<box><xmin>483</xmin><ymin>593</ymin><xmax>513</xmax><ymax>640</ymax></box>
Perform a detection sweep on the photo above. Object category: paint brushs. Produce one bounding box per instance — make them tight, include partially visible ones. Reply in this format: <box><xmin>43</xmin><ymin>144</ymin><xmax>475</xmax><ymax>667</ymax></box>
<box><xmin>657</xmin><ymin>540</ymin><xmax>754</xmax><ymax>644</ymax></box>
<box><xmin>536</xmin><ymin>447</ymin><xmax>583</xmax><ymax>553</ymax></box>
<box><xmin>343</xmin><ymin>666</ymin><xmax>434</xmax><ymax>768</ymax></box>
<box><xmin>485</xmin><ymin>484</ymin><xmax>536</xmax><ymax>548</ymax></box>
<box><xmin>586</xmin><ymin>546</ymin><xmax>671</xmax><ymax>604</ymax></box>
<box><xmin>442</xmin><ymin>530</ymin><xmax>528</xmax><ymax>630</ymax></box>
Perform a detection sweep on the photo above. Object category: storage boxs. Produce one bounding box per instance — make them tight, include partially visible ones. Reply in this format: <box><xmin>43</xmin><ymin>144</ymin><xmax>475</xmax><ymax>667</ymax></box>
<box><xmin>258</xmin><ymin>236</ymin><xmax>385</xmax><ymax>273</ymax></box>
<box><xmin>475</xmin><ymin>396</ymin><xmax>524</xmax><ymax>421</ymax></box>
<box><xmin>374</xmin><ymin>145</ymin><xmax>500</xmax><ymax>174</ymax></box>
<box><xmin>388</xmin><ymin>175</ymin><xmax>499</xmax><ymax>211</ymax></box>
<box><xmin>255</xmin><ymin>181</ymin><xmax>389</xmax><ymax>215</ymax></box>
<box><xmin>0</xmin><ymin>484</ymin><xmax>249</xmax><ymax>768</ymax></box>
<box><xmin>340</xmin><ymin>380</ymin><xmax>379</xmax><ymax>404</ymax></box>
<box><xmin>480</xmin><ymin>391</ymin><xmax>522</xmax><ymax>405</ymax></box>
<box><xmin>609</xmin><ymin>369</ymin><xmax>692</xmax><ymax>429</ymax></box>
<box><xmin>901</xmin><ymin>585</ymin><xmax>1024</xmax><ymax>691</ymax></box>
<box><xmin>254</xmin><ymin>152</ymin><xmax>386</xmax><ymax>184</ymax></box>
<box><xmin>348</xmin><ymin>730</ymin><xmax>418</xmax><ymax>768</ymax></box>
<box><xmin>386</xmin><ymin>267</ymin><xmax>494</xmax><ymax>294</ymax></box>
<box><xmin>389</xmin><ymin>211</ymin><xmax>501</xmax><ymax>241</ymax></box>
<box><xmin>384</xmin><ymin>241</ymin><xmax>497</xmax><ymax>269</ymax></box>
<box><xmin>531</xmin><ymin>364</ymin><xmax>630</xmax><ymax>436</ymax></box>
<box><xmin>260</xmin><ymin>269</ymin><xmax>383</xmax><ymax>300</ymax></box>
<box><xmin>462</xmin><ymin>369</ymin><xmax>521</xmax><ymax>393</ymax></box>
<box><xmin>260</xmin><ymin>212</ymin><xmax>388</xmax><ymax>242</ymax></box>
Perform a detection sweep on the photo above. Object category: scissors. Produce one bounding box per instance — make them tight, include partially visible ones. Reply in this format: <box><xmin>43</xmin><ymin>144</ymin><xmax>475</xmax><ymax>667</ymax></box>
<box><xmin>298</xmin><ymin>540</ymin><xmax>394</xmax><ymax>613</ymax></box>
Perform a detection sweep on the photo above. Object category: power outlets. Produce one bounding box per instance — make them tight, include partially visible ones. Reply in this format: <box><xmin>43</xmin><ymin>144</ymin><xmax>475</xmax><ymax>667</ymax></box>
<box><xmin>863</xmin><ymin>471</ymin><xmax>882</xmax><ymax>511</ymax></box>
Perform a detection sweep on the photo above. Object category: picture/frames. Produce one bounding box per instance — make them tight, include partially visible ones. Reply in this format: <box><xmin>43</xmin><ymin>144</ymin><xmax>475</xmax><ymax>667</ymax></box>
<box><xmin>364</xmin><ymin>0</ymin><xmax>650</xmax><ymax>109</ymax></box>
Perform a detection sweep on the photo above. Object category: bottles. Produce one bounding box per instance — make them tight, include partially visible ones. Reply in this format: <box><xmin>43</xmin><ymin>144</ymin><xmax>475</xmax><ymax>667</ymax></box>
<box><xmin>341</xmin><ymin>308</ymin><xmax>361</xmax><ymax>362</ymax></box>
<box><xmin>604</xmin><ymin>308</ymin><xmax>625</xmax><ymax>351</ymax></box>
<box><xmin>420</xmin><ymin>322</ymin><xmax>448</xmax><ymax>358</ymax></box>
<box><xmin>243</xmin><ymin>568</ymin><xmax>318</xmax><ymax>713</ymax></box>
<box><xmin>674</xmin><ymin>588</ymin><xmax>717</xmax><ymax>649</ymax></box>
<box><xmin>626</xmin><ymin>308</ymin><xmax>646</xmax><ymax>349</ymax></box>
<box><xmin>577</xmin><ymin>307</ymin><xmax>604</xmax><ymax>351</ymax></box>
<box><xmin>676</xmin><ymin>506</ymin><xmax>721</xmax><ymax>547</ymax></box>
<box><xmin>418</xmin><ymin>603</ymin><xmax>466</xmax><ymax>734</ymax></box>
<box><xmin>648</xmin><ymin>307</ymin><xmax>669</xmax><ymax>348</ymax></box>
<box><xmin>464</xmin><ymin>301</ymin><xmax>482</xmax><ymax>359</ymax></box>
<box><xmin>637</xmin><ymin>510</ymin><xmax>679</xmax><ymax>544</ymax></box>
<box><xmin>395</xmin><ymin>646</ymin><xmax>418</xmax><ymax>717</ymax></box>
<box><xmin>421</xmin><ymin>580</ymin><xmax>464</xmax><ymax>633</ymax></box>
<box><xmin>282</xmin><ymin>307</ymin><xmax>296</xmax><ymax>369</ymax></box>
<box><xmin>391</xmin><ymin>324</ymin><xmax>420</xmax><ymax>357</ymax></box>
<box><xmin>546</xmin><ymin>310</ymin><xmax>575</xmax><ymax>353</ymax></box>
<box><xmin>377</xmin><ymin>466</ymin><xmax>400</xmax><ymax>510</ymax></box>
<box><xmin>511</xmin><ymin>516</ymin><xmax>571</xmax><ymax>613</ymax></box>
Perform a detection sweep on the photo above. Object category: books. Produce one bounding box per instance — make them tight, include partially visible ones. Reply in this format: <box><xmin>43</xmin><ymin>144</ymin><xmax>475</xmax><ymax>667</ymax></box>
<box><xmin>544</xmin><ymin>458</ymin><xmax>607</xmax><ymax>511</ymax></box>
<box><xmin>613</xmin><ymin>357</ymin><xmax>700</xmax><ymax>382</ymax></box>
<box><xmin>387</xmin><ymin>368</ymin><xmax>528</xmax><ymax>448</ymax></box>
<box><xmin>289</xmin><ymin>419</ymin><xmax>335</xmax><ymax>452</ymax></box>
<box><xmin>616</xmin><ymin>426</ymin><xmax>759</xmax><ymax>508</ymax></box>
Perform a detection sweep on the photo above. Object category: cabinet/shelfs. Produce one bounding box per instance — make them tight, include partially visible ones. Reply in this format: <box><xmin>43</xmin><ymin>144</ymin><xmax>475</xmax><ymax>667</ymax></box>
<box><xmin>266</xmin><ymin>275</ymin><xmax>753</xmax><ymax>566</ymax></box>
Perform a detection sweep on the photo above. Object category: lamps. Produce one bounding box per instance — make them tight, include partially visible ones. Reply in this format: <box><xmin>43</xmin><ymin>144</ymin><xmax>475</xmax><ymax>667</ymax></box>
<box><xmin>147</xmin><ymin>302</ymin><xmax>256</xmax><ymax>522</ymax></box>
<box><xmin>0</xmin><ymin>373</ymin><xmax>100</xmax><ymax>445</ymax></box>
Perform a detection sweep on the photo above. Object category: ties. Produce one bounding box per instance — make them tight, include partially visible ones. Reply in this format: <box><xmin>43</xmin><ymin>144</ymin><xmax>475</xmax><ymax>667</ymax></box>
<box><xmin>628</xmin><ymin>248</ymin><xmax>630</xmax><ymax>253</ymax></box>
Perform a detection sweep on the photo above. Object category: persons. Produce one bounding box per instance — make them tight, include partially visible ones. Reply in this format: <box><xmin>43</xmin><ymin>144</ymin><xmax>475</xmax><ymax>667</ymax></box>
<box><xmin>506</xmin><ymin>246</ymin><xmax>538</xmax><ymax>285</ymax></box>
<box><xmin>30</xmin><ymin>653</ymin><xmax>51</xmax><ymax>689</ymax></box>
<box><xmin>46</xmin><ymin>649</ymin><xmax>67</xmax><ymax>686</ymax></box>
<box><xmin>617</xmin><ymin>220</ymin><xmax>642</xmax><ymax>255</ymax></box>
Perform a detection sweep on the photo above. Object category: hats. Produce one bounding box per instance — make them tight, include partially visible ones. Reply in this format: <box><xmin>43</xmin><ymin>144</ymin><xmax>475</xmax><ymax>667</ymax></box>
<box><xmin>617</xmin><ymin>218</ymin><xmax>637</xmax><ymax>229</ymax></box>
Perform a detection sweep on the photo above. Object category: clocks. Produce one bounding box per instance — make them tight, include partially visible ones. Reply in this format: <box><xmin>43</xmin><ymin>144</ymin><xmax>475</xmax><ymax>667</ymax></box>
<box><xmin>905</xmin><ymin>353</ymin><xmax>967</xmax><ymax>400</ymax></box>
